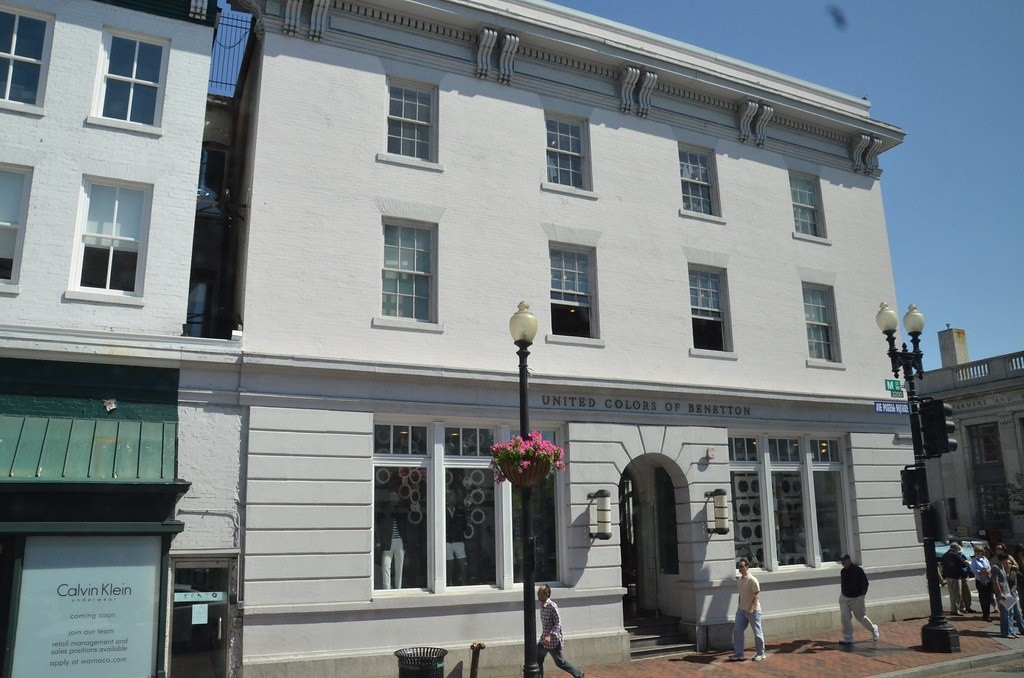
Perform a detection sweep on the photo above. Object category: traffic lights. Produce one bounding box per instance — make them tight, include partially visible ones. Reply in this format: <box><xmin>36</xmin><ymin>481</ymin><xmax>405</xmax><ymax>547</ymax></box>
<box><xmin>919</xmin><ymin>400</ymin><xmax>958</xmax><ymax>453</ymax></box>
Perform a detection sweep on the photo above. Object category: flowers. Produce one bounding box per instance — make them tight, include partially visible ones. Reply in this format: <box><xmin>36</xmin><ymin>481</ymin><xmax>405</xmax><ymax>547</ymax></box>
<box><xmin>490</xmin><ymin>429</ymin><xmax>566</xmax><ymax>485</ymax></box>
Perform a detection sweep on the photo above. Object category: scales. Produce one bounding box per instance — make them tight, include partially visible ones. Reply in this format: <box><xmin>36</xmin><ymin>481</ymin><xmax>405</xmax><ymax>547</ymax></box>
<box><xmin>394</xmin><ymin>647</ymin><xmax>448</xmax><ymax>678</ymax></box>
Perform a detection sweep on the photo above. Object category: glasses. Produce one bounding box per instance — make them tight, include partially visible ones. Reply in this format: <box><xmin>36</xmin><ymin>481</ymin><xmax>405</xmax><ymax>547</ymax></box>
<box><xmin>738</xmin><ymin>565</ymin><xmax>744</xmax><ymax>567</ymax></box>
<box><xmin>994</xmin><ymin>547</ymin><xmax>1003</xmax><ymax>550</ymax></box>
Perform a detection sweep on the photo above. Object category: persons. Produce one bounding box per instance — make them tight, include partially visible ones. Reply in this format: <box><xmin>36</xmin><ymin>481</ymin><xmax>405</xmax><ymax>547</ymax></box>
<box><xmin>376</xmin><ymin>492</ymin><xmax>405</xmax><ymax>589</ymax></box>
<box><xmin>729</xmin><ymin>559</ymin><xmax>765</xmax><ymax>661</ymax></box>
<box><xmin>446</xmin><ymin>492</ymin><xmax>467</xmax><ymax>585</ymax></box>
<box><xmin>537</xmin><ymin>584</ymin><xmax>584</xmax><ymax>678</ymax></box>
<box><xmin>938</xmin><ymin>542</ymin><xmax>1024</xmax><ymax>638</ymax></box>
<box><xmin>838</xmin><ymin>554</ymin><xmax>879</xmax><ymax>643</ymax></box>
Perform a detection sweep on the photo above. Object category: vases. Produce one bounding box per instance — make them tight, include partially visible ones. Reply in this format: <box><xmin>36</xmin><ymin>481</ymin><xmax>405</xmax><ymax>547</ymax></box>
<box><xmin>500</xmin><ymin>458</ymin><xmax>550</xmax><ymax>487</ymax></box>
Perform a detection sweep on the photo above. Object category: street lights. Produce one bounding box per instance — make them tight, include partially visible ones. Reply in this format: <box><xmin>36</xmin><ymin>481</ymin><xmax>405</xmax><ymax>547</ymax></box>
<box><xmin>877</xmin><ymin>302</ymin><xmax>961</xmax><ymax>653</ymax></box>
<box><xmin>510</xmin><ymin>301</ymin><xmax>540</xmax><ymax>678</ymax></box>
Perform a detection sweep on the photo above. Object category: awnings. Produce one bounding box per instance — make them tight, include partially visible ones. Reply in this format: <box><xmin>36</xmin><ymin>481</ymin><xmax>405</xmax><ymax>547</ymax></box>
<box><xmin>2</xmin><ymin>360</ymin><xmax>192</xmax><ymax>519</ymax></box>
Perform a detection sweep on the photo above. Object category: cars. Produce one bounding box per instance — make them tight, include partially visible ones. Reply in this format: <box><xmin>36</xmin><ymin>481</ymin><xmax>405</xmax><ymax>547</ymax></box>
<box><xmin>934</xmin><ymin>535</ymin><xmax>990</xmax><ymax>580</ymax></box>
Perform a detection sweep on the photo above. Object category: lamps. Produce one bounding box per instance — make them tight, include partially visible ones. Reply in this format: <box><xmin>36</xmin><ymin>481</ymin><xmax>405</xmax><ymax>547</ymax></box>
<box><xmin>704</xmin><ymin>489</ymin><xmax>729</xmax><ymax>535</ymax></box>
<box><xmin>587</xmin><ymin>489</ymin><xmax>612</xmax><ymax>541</ymax></box>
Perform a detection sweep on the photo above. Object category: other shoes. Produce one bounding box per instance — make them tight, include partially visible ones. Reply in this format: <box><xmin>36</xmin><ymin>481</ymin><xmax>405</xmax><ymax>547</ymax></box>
<box><xmin>941</xmin><ymin>582</ymin><xmax>948</xmax><ymax>587</ymax></box>
<box><xmin>1002</xmin><ymin>631</ymin><xmax>1019</xmax><ymax>639</ymax></box>
<box><xmin>967</xmin><ymin>609</ymin><xmax>976</xmax><ymax>613</ymax></box>
<box><xmin>982</xmin><ymin>616</ymin><xmax>992</xmax><ymax>622</ymax></box>
<box><xmin>871</xmin><ymin>624</ymin><xmax>879</xmax><ymax>641</ymax></box>
<box><xmin>951</xmin><ymin>611</ymin><xmax>964</xmax><ymax>616</ymax></box>
<box><xmin>961</xmin><ymin>609</ymin><xmax>968</xmax><ymax>613</ymax></box>
<box><xmin>839</xmin><ymin>637</ymin><xmax>855</xmax><ymax>644</ymax></box>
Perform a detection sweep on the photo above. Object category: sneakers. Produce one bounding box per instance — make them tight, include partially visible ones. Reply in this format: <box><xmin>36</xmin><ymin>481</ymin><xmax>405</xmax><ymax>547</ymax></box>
<box><xmin>729</xmin><ymin>654</ymin><xmax>744</xmax><ymax>660</ymax></box>
<box><xmin>752</xmin><ymin>653</ymin><xmax>765</xmax><ymax>661</ymax></box>
